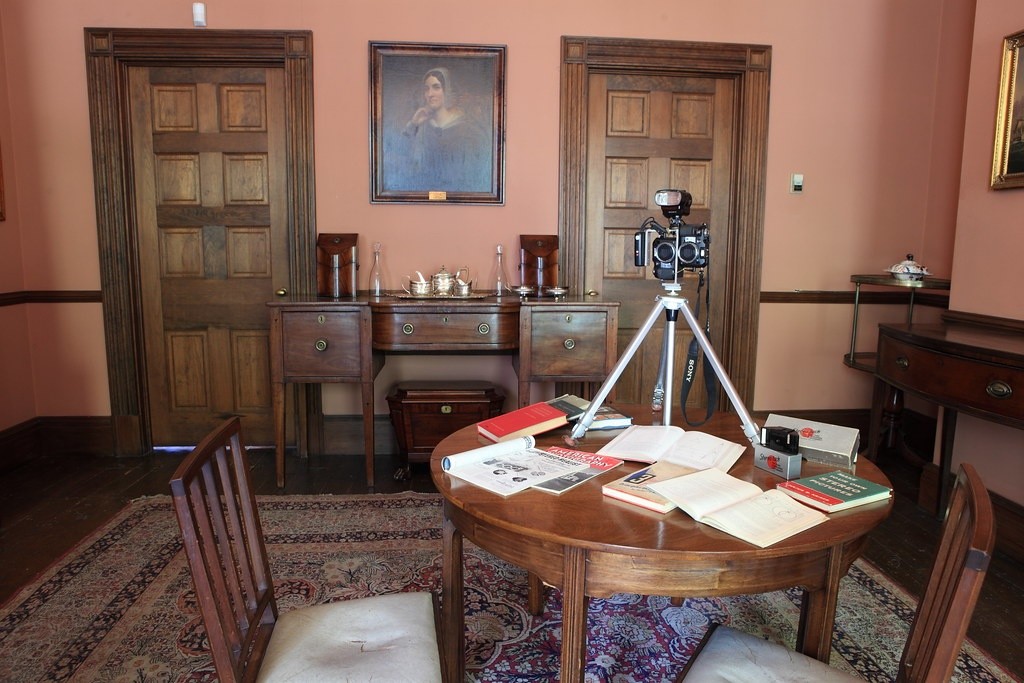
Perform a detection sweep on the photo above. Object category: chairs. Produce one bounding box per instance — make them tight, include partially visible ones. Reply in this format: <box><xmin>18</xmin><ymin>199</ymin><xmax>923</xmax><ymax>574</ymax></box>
<box><xmin>683</xmin><ymin>462</ymin><xmax>995</xmax><ymax>683</ymax></box>
<box><xmin>167</xmin><ymin>416</ymin><xmax>448</xmax><ymax>683</ymax></box>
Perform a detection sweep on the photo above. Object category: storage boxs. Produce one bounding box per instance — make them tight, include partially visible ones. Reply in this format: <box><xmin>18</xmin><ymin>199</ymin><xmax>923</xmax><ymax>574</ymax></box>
<box><xmin>764</xmin><ymin>413</ymin><xmax>861</xmax><ymax>468</ymax></box>
<box><xmin>753</xmin><ymin>443</ymin><xmax>802</xmax><ymax>480</ymax></box>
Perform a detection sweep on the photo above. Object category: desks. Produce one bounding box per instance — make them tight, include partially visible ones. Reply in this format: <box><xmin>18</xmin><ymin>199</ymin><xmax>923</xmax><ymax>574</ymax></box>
<box><xmin>429</xmin><ymin>402</ymin><xmax>895</xmax><ymax>683</ymax></box>
<box><xmin>867</xmin><ymin>319</ymin><xmax>1024</xmax><ymax>522</ymax></box>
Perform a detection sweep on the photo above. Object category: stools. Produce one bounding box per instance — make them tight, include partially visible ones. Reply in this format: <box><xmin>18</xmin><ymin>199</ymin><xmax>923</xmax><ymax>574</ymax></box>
<box><xmin>384</xmin><ymin>380</ymin><xmax>508</xmax><ymax>484</ymax></box>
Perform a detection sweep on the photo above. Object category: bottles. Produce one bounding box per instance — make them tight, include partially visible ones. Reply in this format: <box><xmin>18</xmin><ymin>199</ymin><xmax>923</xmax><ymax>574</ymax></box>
<box><xmin>368</xmin><ymin>241</ymin><xmax>385</xmax><ymax>297</ymax></box>
<box><xmin>492</xmin><ymin>244</ymin><xmax>508</xmax><ymax>297</ymax></box>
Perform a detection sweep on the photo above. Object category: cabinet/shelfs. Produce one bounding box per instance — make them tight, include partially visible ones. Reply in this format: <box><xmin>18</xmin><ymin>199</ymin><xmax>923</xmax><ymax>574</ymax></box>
<box><xmin>845</xmin><ymin>274</ymin><xmax>952</xmax><ymax>469</ymax></box>
<box><xmin>265</xmin><ymin>289</ymin><xmax>621</xmax><ymax>489</ymax></box>
<box><xmin>0</xmin><ymin>490</ymin><xmax>1023</xmax><ymax>683</ymax></box>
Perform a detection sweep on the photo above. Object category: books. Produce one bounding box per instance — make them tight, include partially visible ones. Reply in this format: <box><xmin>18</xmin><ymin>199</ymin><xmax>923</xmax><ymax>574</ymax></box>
<box><xmin>441</xmin><ymin>435</ymin><xmax>590</xmax><ymax>497</ymax></box>
<box><xmin>595</xmin><ymin>424</ymin><xmax>747</xmax><ymax>474</ymax></box>
<box><xmin>602</xmin><ymin>460</ymin><xmax>701</xmax><ymax>514</ymax></box>
<box><xmin>646</xmin><ymin>467</ymin><xmax>830</xmax><ymax>548</ymax></box>
<box><xmin>776</xmin><ymin>470</ymin><xmax>893</xmax><ymax>514</ymax></box>
<box><xmin>532</xmin><ymin>446</ymin><xmax>624</xmax><ymax>495</ymax></box>
<box><xmin>477</xmin><ymin>393</ymin><xmax>633</xmax><ymax>444</ymax></box>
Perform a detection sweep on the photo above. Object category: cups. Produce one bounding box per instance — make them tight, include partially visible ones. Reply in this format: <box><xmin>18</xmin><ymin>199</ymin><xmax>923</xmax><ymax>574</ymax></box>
<box><xmin>401</xmin><ymin>270</ymin><xmax>431</xmax><ymax>296</ymax></box>
<box><xmin>453</xmin><ymin>279</ymin><xmax>472</xmax><ymax>297</ymax></box>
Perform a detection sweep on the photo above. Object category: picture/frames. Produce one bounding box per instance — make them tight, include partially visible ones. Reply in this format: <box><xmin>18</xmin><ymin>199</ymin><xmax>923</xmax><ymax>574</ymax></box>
<box><xmin>989</xmin><ymin>29</ymin><xmax>1024</xmax><ymax>189</ymax></box>
<box><xmin>368</xmin><ymin>40</ymin><xmax>507</xmax><ymax>206</ymax></box>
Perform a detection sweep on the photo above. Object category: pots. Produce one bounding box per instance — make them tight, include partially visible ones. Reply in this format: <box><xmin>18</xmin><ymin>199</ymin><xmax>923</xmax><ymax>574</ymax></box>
<box><xmin>884</xmin><ymin>253</ymin><xmax>933</xmax><ymax>282</ymax></box>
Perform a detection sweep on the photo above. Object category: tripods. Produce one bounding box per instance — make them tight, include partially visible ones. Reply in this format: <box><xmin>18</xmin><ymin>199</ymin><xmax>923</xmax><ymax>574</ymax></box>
<box><xmin>571</xmin><ymin>283</ymin><xmax>761</xmax><ymax>449</ymax></box>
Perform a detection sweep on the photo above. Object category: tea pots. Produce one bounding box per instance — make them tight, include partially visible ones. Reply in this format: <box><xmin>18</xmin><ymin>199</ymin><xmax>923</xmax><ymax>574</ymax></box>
<box><xmin>430</xmin><ymin>265</ymin><xmax>469</xmax><ymax>296</ymax></box>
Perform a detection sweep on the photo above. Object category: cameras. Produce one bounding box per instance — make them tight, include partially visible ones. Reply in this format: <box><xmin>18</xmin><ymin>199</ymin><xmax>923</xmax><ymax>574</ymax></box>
<box><xmin>635</xmin><ymin>190</ymin><xmax>711</xmax><ymax>281</ymax></box>
